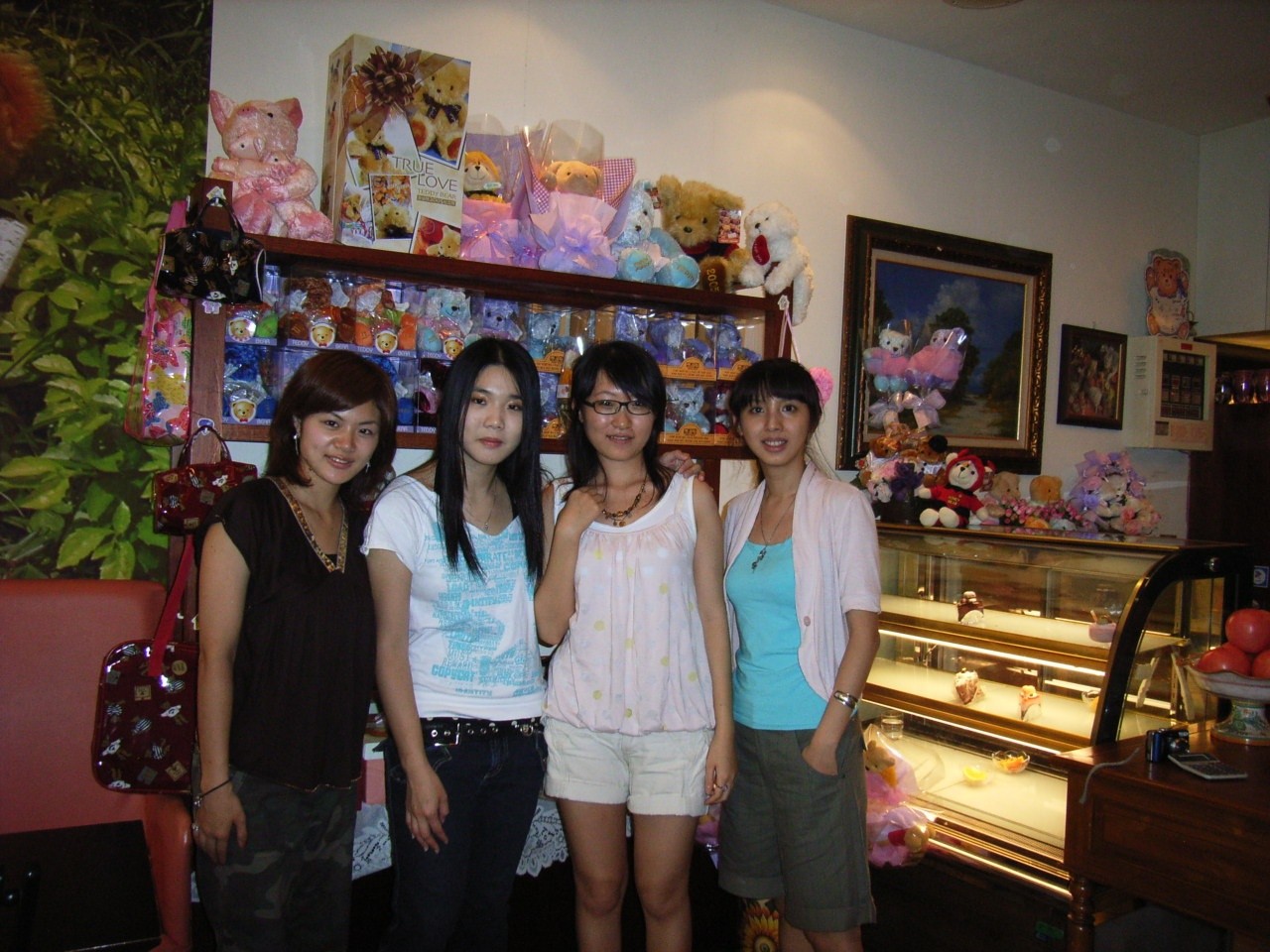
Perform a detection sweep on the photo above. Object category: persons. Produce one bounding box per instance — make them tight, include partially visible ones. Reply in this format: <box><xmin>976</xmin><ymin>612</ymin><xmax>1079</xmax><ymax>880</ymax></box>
<box><xmin>719</xmin><ymin>357</ymin><xmax>882</xmax><ymax>952</ymax></box>
<box><xmin>359</xmin><ymin>336</ymin><xmax>705</xmax><ymax>952</ymax></box>
<box><xmin>190</xmin><ymin>349</ymin><xmax>398</xmax><ymax>952</ymax></box>
<box><xmin>534</xmin><ymin>340</ymin><xmax>740</xmax><ymax>952</ymax></box>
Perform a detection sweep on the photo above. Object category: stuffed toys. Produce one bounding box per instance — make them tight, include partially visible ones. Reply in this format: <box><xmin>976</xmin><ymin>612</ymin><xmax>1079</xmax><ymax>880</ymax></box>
<box><xmin>340</xmin><ymin>50</ymin><xmax>470</xmax><ymax>260</ymax></box>
<box><xmin>210</xmin><ymin>89</ymin><xmax>335</xmax><ymax>243</ymax></box>
<box><xmin>539</xmin><ymin>161</ymin><xmax>602</xmax><ymax>198</ymax></box>
<box><xmin>854</xmin><ymin>319</ymin><xmax>1161</xmax><ymax>536</ymax></box>
<box><xmin>463</xmin><ymin>150</ymin><xmax>505</xmax><ymax>204</ymax></box>
<box><xmin>656</xmin><ymin>174</ymin><xmax>751</xmax><ymax>295</ymax></box>
<box><xmin>738</xmin><ymin>200</ymin><xmax>815</xmax><ymax>326</ymax></box>
<box><xmin>611</xmin><ymin>178</ymin><xmax>700</xmax><ymax>289</ymax></box>
<box><xmin>223</xmin><ymin>271</ymin><xmax>765</xmax><ymax>442</ymax></box>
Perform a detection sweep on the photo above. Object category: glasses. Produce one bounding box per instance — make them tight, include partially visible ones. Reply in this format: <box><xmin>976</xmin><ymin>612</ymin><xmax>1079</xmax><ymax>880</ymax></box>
<box><xmin>582</xmin><ymin>397</ymin><xmax>651</xmax><ymax>415</ymax></box>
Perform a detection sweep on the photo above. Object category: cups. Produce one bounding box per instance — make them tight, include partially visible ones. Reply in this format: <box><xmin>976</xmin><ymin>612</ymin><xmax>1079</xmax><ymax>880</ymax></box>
<box><xmin>880</xmin><ymin>710</ymin><xmax>905</xmax><ymax>740</ymax></box>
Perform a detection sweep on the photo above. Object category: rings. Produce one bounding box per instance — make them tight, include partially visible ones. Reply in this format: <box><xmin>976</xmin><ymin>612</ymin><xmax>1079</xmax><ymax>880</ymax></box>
<box><xmin>191</xmin><ymin>823</ymin><xmax>199</xmax><ymax>831</ymax></box>
<box><xmin>716</xmin><ymin>783</ymin><xmax>728</xmax><ymax>792</ymax></box>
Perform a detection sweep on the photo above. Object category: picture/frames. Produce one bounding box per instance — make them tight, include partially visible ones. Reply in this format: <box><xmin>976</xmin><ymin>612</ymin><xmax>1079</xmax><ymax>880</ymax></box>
<box><xmin>834</xmin><ymin>214</ymin><xmax>1053</xmax><ymax>476</ymax></box>
<box><xmin>1055</xmin><ymin>324</ymin><xmax>1128</xmax><ymax>431</ymax></box>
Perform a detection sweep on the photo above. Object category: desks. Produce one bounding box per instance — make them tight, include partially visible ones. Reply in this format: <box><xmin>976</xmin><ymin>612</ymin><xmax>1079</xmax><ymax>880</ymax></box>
<box><xmin>351</xmin><ymin>724</ymin><xmax>635</xmax><ymax>952</ymax></box>
<box><xmin>0</xmin><ymin>820</ymin><xmax>162</xmax><ymax>952</ymax></box>
<box><xmin>1046</xmin><ymin>719</ymin><xmax>1270</xmax><ymax>952</ymax></box>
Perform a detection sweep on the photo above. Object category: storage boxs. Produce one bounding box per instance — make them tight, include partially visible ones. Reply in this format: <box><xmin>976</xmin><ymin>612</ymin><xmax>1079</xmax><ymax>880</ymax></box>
<box><xmin>221</xmin><ymin>263</ymin><xmax>764</xmax><ymax>445</ymax></box>
<box><xmin>316</xmin><ymin>34</ymin><xmax>473</xmax><ymax>259</ymax></box>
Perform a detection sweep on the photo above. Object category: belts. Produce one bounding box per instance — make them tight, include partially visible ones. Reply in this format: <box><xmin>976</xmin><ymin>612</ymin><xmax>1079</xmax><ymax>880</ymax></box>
<box><xmin>420</xmin><ymin>718</ymin><xmax>542</xmax><ymax>746</ymax></box>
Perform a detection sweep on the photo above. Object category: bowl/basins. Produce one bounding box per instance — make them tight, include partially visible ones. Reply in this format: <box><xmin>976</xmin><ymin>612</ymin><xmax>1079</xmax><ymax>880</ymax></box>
<box><xmin>1082</xmin><ymin>689</ymin><xmax>1100</xmax><ymax>707</ymax></box>
<box><xmin>991</xmin><ymin>750</ymin><xmax>1030</xmax><ymax>773</ymax></box>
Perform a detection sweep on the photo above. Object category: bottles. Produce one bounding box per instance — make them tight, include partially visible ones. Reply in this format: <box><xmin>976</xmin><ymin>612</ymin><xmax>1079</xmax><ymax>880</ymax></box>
<box><xmin>1214</xmin><ymin>371</ymin><xmax>1233</xmax><ymax>404</ymax></box>
<box><xmin>1232</xmin><ymin>370</ymin><xmax>1256</xmax><ymax>403</ymax></box>
<box><xmin>1256</xmin><ymin>369</ymin><xmax>1270</xmax><ymax>402</ymax></box>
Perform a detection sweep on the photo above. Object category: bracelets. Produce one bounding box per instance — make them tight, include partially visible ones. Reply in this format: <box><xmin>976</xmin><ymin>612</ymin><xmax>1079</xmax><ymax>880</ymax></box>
<box><xmin>194</xmin><ymin>777</ymin><xmax>234</xmax><ymax>807</ymax></box>
<box><xmin>831</xmin><ymin>689</ymin><xmax>859</xmax><ymax>710</ymax></box>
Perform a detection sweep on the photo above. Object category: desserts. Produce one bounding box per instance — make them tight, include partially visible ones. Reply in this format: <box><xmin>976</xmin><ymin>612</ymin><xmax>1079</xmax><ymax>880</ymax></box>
<box><xmin>1018</xmin><ymin>685</ymin><xmax>1041</xmax><ymax>720</ymax></box>
<box><xmin>1088</xmin><ymin>610</ymin><xmax>1117</xmax><ymax>642</ymax></box>
<box><xmin>957</xmin><ymin>591</ymin><xmax>984</xmax><ymax>624</ymax></box>
<box><xmin>954</xmin><ymin>668</ymin><xmax>978</xmax><ymax>703</ymax></box>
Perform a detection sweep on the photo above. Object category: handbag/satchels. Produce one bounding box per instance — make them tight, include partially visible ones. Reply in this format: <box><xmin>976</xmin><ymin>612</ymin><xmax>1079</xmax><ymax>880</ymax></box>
<box><xmin>122</xmin><ymin>227</ymin><xmax>192</xmax><ymax>447</ymax></box>
<box><xmin>157</xmin><ymin>195</ymin><xmax>262</xmax><ymax>308</ymax></box>
<box><xmin>88</xmin><ymin>536</ymin><xmax>205</xmax><ymax>793</ymax></box>
<box><xmin>152</xmin><ymin>425</ymin><xmax>258</xmax><ymax>533</ymax></box>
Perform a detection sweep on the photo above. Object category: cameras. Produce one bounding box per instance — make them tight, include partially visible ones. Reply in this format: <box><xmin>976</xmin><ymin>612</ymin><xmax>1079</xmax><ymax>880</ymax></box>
<box><xmin>1145</xmin><ymin>728</ymin><xmax>1192</xmax><ymax>763</ymax></box>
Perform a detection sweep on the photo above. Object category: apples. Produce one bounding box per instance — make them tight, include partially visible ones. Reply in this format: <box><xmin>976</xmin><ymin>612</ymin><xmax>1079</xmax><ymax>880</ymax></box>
<box><xmin>1195</xmin><ymin>601</ymin><xmax>1270</xmax><ymax>681</ymax></box>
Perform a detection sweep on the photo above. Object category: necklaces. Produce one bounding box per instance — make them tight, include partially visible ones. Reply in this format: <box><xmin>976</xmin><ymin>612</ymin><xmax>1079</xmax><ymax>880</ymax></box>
<box><xmin>602</xmin><ymin>471</ymin><xmax>647</xmax><ymax>526</ymax></box>
<box><xmin>462</xmin><ymin>476</ymin><xmax>497</xmax><ymax>531</ymax></box>
<box><xmin>752</xmin><ymin>495</ymin><xmax>795</xmax><ymax>574</ymax></box>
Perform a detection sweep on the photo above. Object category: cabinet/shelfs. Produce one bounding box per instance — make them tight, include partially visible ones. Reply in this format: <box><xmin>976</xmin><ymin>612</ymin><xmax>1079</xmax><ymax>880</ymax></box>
<box><xmin>170</xmin><ymin>177</ymin><xmax>794</xmax><ymax>642</ymax></box>
<box><xmin>857</xmin><ymin>520</ymin><xmax>1255</xmax><ymax>952</ymax></box>
